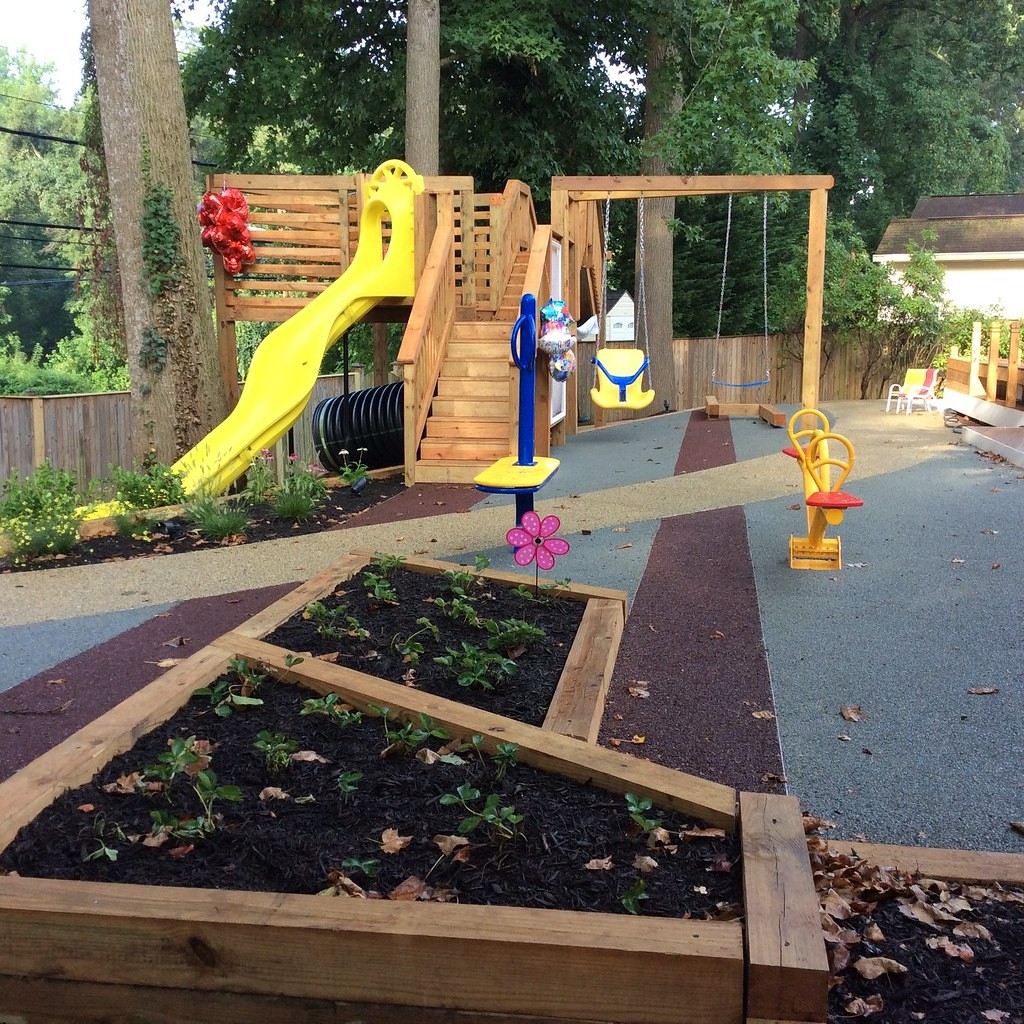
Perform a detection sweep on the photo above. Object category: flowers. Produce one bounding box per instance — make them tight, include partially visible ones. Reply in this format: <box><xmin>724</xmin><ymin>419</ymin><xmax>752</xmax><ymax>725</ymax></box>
<box><xmin>0</xmin><ymin>442</ymin><xmax>367</xmax><ymax>551</ymax></box>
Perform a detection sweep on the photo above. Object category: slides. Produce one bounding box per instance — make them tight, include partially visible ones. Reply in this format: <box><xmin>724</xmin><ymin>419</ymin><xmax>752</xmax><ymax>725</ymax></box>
<box><xmin>65</xmin><ymin>259</ymin><xmax>416</xmax><ymax>521</ymax></box>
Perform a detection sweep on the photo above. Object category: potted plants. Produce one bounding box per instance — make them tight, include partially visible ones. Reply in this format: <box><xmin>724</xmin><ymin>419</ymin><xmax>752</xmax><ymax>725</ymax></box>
<box><xmin>767</xmin><ymin>785</ymin><xmax>1023</xmax><ymax>1024</ymax></box>
<box><xmin>0</xmin><ymin>636</ymin><xmax>781</xmax><ymax>1024</ymax></box>
<box><xmin>222</xmin><ymin>546</ymin><xmax>631</xmax><ymax>745</ymax></box>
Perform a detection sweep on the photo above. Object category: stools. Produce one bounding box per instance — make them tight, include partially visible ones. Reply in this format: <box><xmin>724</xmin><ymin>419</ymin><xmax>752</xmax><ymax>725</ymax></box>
<box><xmin>473</xmin><ymin>452</ymin><xmax>560</xmax><ymax>495</ymax></box>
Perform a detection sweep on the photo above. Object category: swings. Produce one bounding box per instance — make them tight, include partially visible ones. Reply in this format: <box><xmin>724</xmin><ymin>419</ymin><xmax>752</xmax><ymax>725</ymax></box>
<box><xmin>590</xmin><ymin>194</ymin><xmax>656</xmax><ymax>411</ymax></box>
<box><xmin>711</xmin><ymin>191</ymin><xmax>770</xmax><ymax>387</ymax></box>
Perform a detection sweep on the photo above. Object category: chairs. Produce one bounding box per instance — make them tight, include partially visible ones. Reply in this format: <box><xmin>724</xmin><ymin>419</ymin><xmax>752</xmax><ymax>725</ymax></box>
<box><xmin>782</xmin><ymin>409</ymin><xmax>829</xmax><ymax>461</ymax></box>
<box><xmin>805</xmin><ymin>434</ymin><xmax>866</xmax><ymax>508</ymax></box>
<box><xmin>886</xmin><ymin>369</ymin><xmax>943</xmax><ymax>415</ymax></box>
<box><xmin>587</xmin><ymin>347</ymin><xmax>656</xmax><ymax>409</ymax></box>
